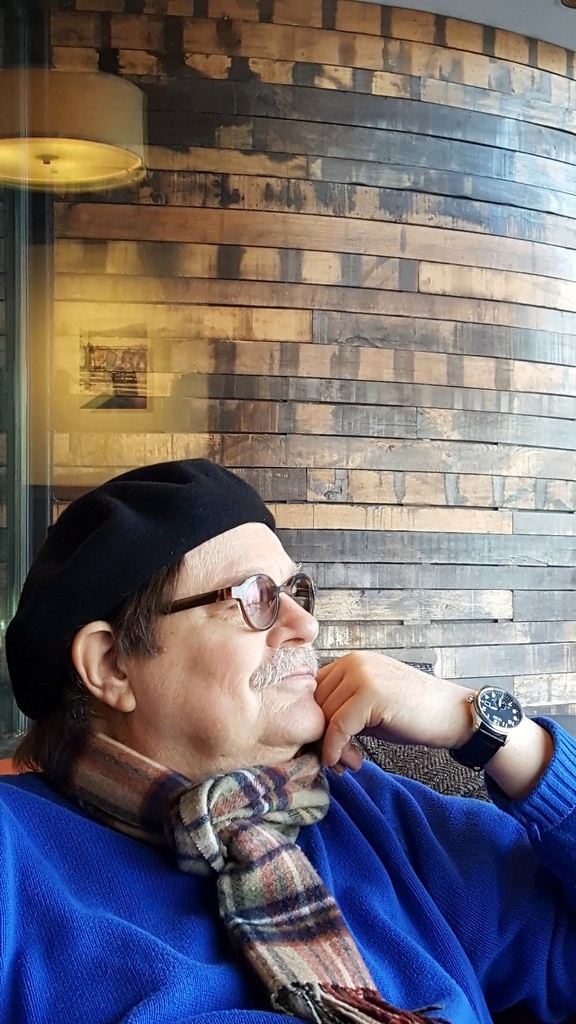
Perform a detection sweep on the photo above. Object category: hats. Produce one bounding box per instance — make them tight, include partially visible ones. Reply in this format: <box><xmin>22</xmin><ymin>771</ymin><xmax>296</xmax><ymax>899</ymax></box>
<box><xmin>5</xmin><ymin>458</ymin><xmax>276</xmax><ymax>721</ymax></box>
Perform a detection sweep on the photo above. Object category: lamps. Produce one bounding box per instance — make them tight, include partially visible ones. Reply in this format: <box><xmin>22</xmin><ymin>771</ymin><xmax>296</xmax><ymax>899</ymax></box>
<box><xmin>0</xmin><ymin>69</ymin><xmax>148</xmax><ymax>193</ymax></box>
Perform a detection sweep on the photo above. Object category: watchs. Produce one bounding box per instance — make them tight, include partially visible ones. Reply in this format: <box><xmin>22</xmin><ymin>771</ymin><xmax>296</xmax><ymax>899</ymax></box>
<box><xmin>447</xmin><ymin>685</ymin><xmax>525</xmax><ymax>772</ymax></box>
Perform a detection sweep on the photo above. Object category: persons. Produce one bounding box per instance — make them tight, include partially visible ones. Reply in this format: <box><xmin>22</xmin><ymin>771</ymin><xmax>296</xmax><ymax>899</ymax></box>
<box><xmin>0</xmin><ymin>456</ymin><xmax>576</xmax><ymax>1023</ymax></box>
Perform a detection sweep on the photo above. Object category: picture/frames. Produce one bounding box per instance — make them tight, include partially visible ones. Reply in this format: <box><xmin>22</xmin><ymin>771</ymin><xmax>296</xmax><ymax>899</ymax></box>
<box><xmin>60</xmin><ymin>303</ymin><xmax>170</xmax><ymax>431</ymax></box>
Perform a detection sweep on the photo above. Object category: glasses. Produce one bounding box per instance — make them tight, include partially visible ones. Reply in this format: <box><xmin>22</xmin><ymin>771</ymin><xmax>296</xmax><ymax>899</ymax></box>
<box><xmin>153</xmin><ymin>573</ymin><xmax>315</xmax><ymax>631</ymax></box>
